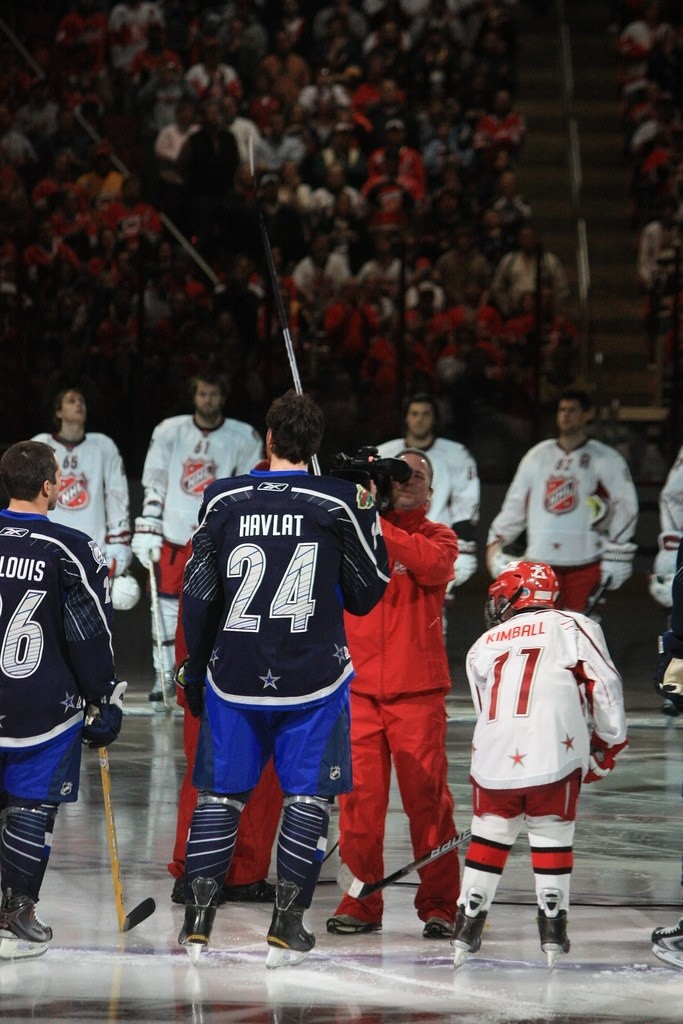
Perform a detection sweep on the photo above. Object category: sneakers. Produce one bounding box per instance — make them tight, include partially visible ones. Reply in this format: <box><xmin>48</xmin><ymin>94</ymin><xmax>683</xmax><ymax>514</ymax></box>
<box><xmin>661</xmin><ymin>697</ymin><xmax>681</xmax><ymax>726</ymax></box>
<box><xmin>266</xmin><ymin>880</ymin><xmax>315</xmax><ymax>970</ymax></box>
<box><xmin>222</xmin><ymin>877</ymin><xmax>276</xmax><ymax>903</ymax></box>
<box><xmin>327</xmin><ymin>912</ymin><xmax>382</xmax><ymax>934</ymax></box>
<box><xmin>178</xmin><ymin>876</ymin><xmax>219</xmax><ymax>961</ymax></box>
<box><xmin>171</xmin><ymin>877</ymin><xmax>226</xmax><ymax>905</ymax></box>
<box><xmin>0</xmin><ymin>871</ymin><xmax>53</xmax><ymax>960</ymax></box>
<box><xmin>450</xmin><ymin>888</ymin><xmax>487</xmax><ymax>970</ymax></box>
<box><xmin>538</xmin><ymin>889</ymin><xmax>570</xmax><ymax>968</ymax></box>
<box><xmin>652</xmin><ymin>917</ymin><xmax>683</xmax><ymax>969</ymax></box>
<box><xmin>423</xmin><ymin>916</ymin><xmax>454</xmax><ymax>938</ymax></box>
<box><xmin>149</xmin><ymin>671</ymin><xmax>175</xmax><ymax>712</ymax></box>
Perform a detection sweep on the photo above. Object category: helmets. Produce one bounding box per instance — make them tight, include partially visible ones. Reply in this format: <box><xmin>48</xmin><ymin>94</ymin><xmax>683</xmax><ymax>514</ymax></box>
<box><xmin>486</xmin><ymin>561</ymin><xmax>559</xmax><ymax>628</ymax></box>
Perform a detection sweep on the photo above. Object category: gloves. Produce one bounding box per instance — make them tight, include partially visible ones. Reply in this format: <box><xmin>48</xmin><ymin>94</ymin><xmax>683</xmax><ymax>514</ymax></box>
<box><xmin>485</xmin><ymin>530</ymin><xmax>520</xmax><ymax>579</ymax></box>
<box><xmin>582</xmin><ymin>727</ymin><xmax>630</xmax><ymax>783</ymax></box>
<box><xmin>104</xmin><ymin>526</ymin><xmax>133</xmax><ymax>575</ymax></box>
<box><xmin>453</xmin><ymin>539</ymin><xmax>478</xmax><ymax>587</ymax></box>
<box><xmin>183</xmin><ymin>675</ymin><xmax>203</xmax><ymax>717</ymax></box>
<box><xmin>654</xmin><ymin>530</ymin><xmax>682</xmax><ymax>574</ymax></box>
<box><xmin>653</xmin><ymin>631</ymin><xmax>683</xmax><ymax>700</ymax></box>
<box><xmin>80</xmin><ymin>679</ymin><xmax>128</xmax><ymax>749</ymax></box>
<box><xmin>599</xmin><ymin>543</ymin><xmax>638</xmax><ymax>590</ymax></box>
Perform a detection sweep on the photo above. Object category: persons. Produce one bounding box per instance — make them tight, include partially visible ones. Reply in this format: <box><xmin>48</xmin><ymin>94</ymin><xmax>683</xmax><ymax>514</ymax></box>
<box><xmin>29</xmin><ymin>390</ymin><xmax>140</xmax><ymax>612</ymax></box>
<box><xmin>325</xmin><ymin>445</ymin><xmax>460</xmax><ymax>937</ymax></box>
<box><xmin>449</xmin><ymin>561</ymin><xmax>629</xmax><ymax>968</ymax></box>
<box><xmin>373</xmin><ymin>393</ymin><xmax>483</xmax><ymax>649</ymax></box>
<box><xmin>610</xmin><ymin>0</ymin><xmax>683</xmax><ymax>407</ymax></box>
<box><xmin>646</xmin><ymin>444</ymin><xmax>683</xmax><ymax>715</ymax></box>
<box><xmin>0</xmin><ymin>441</ymin><xmax>123</xmax><ymax>961</ymax></box>
<box><xmin>485</xmin><ymin>386</ymin><xmax>638</xmax><ymax>615</ymax></box>
<box><xmin>176</xmin><ymin>389</ymin><xmax>389</xmax><ymax>972</ymax></box>
<box><xmin>1</xmin><ymin>1</ymin><xmax>602</xmax><ymax>421</ymax></box>
<box><xmin>132</xmin><ymin>370</ymin><xmax>262</xmax><ymax>714</ymax></box>
<box><xmin>169</xmin><ymin>593</ymin><xmax>285</xmax><ymax>902</ymax></box>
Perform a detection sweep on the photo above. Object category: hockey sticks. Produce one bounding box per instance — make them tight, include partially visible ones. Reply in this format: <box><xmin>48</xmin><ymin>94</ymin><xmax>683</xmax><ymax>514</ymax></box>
<box><xmin>87</xmin><ymin>703</ymin><xmax>156</xmax><ymax>934</ymax></box>
<box><xmin>582</xmin><ymin>574</ymin><xmax>612</xmax><ymax>618</ymax></box>
<box><xmin>108</xmin><ymin>558</ymin><xmax>116</xmax><ymax>597</ymax></box>
<box><xmin>335</xmin><ymin>750</ymin><xmax>606</xmax><ymax>900</ymax></box>
<box><xmin>147</xmin><ymin>548</ymin><xmax>185</xmax><ymax>722</ymax></box>
<box><xmin>248</xmin><ymin>136</ymin><xmax>322</xmax><ymax>474</ymax></box>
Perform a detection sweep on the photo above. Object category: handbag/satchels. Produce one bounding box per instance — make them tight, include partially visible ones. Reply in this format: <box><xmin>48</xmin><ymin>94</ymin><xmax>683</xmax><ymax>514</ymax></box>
<box><xmin>130</xmin><ymin>516</ymin><xmax>164</xmax><ymax>569</ymax></box>
